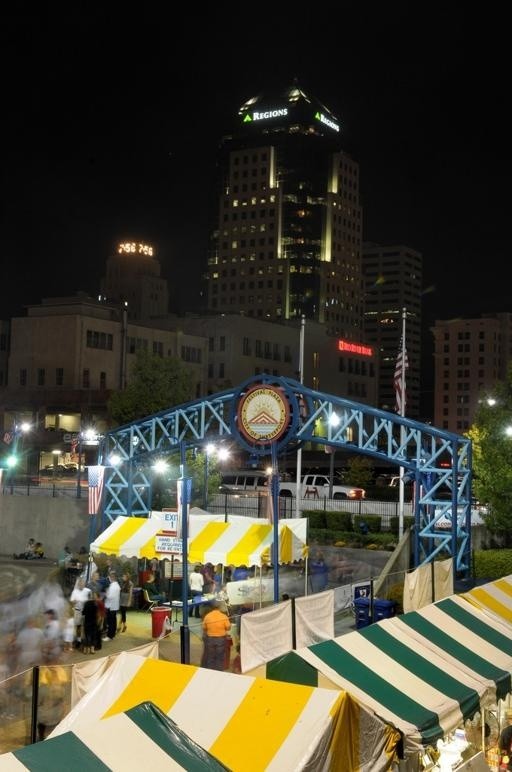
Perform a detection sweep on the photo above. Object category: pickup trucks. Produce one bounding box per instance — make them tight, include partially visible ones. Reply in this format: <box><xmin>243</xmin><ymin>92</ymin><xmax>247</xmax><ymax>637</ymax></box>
<box><xmin>278</xmin><ymin>474</ymin><xmax>366</xmax><ymax>500</ymax></box>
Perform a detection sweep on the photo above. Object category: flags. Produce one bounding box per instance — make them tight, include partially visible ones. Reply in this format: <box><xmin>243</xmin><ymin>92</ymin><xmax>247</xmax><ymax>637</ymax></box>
<box><xmin>85</xmin><ymin>466</ymin><xmax>107</xmax><ymax>516</ymax></box>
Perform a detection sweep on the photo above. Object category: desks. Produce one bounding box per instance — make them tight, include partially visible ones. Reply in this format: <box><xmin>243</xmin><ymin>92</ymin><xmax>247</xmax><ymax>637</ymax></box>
<box><xmin>162</xmin><ymin>593</ymin><xmax>217</xmax><ymax>623</ymax></box>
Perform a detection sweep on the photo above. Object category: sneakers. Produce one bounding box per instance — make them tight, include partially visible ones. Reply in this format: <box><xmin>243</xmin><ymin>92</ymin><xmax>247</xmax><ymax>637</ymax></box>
<box><xmin>102</xmin><ymin>625</ymin><xmax>128</xmax><ymax>641</ymax></box>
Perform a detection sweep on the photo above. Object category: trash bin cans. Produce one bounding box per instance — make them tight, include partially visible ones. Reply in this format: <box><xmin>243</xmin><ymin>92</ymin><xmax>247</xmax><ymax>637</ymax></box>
<box><xmin>353</xmin><ymin>597</ymin><xmax>398</xmax><ymax>628</ymax></box>
<box><xmin>150</xmin><ymin>606</ymin><xmax>172</xmax><ymax>638</ymax></box>
<box><xmin>215</xmin><ymin>637</ymin><xmax>233</xmax><ymax>671</ymax></box>
<box><xmin>311</xmin><ymin>562</ymin><xmax>328</xmax><ymax>593</ymax></box>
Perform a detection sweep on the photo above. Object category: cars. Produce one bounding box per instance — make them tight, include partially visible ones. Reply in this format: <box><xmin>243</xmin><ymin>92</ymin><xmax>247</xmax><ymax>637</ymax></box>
<box><xmin>374</xmin><ymin>473</ymin><xmax>475</xmax><ymax>488</ymax></box>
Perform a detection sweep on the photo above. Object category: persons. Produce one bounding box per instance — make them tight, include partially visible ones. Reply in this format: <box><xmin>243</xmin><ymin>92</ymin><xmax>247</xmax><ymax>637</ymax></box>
<box><xmin>0</xmin><ymin>533</ymin><xmax>168</xmax><ymax>741</ymax></box>
<box><xmin>232</xmin><ymin>566</ymin><xmax>250</xmax><ymax>613</ymax></box>
<box><xmin>188</xmin><ymin>564</ymin><xmax>201</xmax><ymax>618</ymax></box>
<box><xmin>198</xmin><ymin>563</ymin><xmax>216</xmax><ymax>604</ymax></box>
<box><xmin>496</xmin><ymin>723</ymin><xmax>511</xmax><ymax>771</ymax></box>
<box><xmin>201</xmin><ymin>603</ymin><xmax>231</xmax><ymax>671</ymax></box>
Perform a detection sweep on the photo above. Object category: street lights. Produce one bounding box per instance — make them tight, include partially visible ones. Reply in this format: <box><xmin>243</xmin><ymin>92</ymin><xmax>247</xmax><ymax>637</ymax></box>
<box><xmin>153</xmin><ymin>435</ymin><xmax>190</xmax><ymax>664</ymax></box>
<box><xmin>77</xmin><ymin>429</ymin><xmax>98</xmax><ymax>498</ymax></box>
<box><xmin>109</xmin><ymin>454</ymin><xmax>134</xmax><ymax>517</ymax></box>
<box><xmin>205</xmin><ymin>428</ymin><xmax>279</xmax><ymax>603</ymax></box>
<box><xmin>329</xmin><ymin>410</ymin><xmax>340</xmax><ymax>500</ymax></box>
<box><xmin>10</xmin><ymin>422</ymin><xmax>31</xmax><ymax>495</ymax></box>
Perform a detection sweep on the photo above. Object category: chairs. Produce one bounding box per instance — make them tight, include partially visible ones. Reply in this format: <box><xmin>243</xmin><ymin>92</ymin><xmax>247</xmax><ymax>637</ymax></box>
<box><xmin>141</xmin><ymin>588</ymin><xmax>160</xmax><ymax>614</ymax></box>
